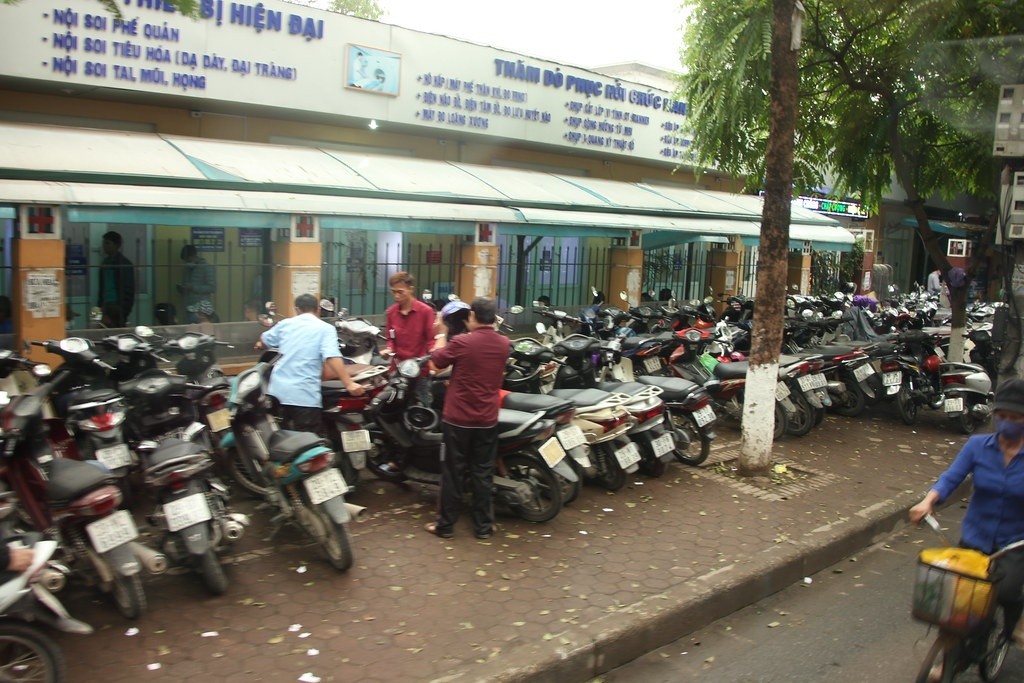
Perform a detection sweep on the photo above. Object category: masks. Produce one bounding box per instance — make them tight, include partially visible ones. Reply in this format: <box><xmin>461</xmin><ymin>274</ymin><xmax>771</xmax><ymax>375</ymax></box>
<box><xmin>993</xmin><ymin>417</ymin><xmax>1024</xmax><ymax>441</ymax></box>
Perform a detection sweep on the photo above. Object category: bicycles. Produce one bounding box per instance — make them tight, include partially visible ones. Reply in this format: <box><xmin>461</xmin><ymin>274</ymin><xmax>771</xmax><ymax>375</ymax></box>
<box><xmin>906</xmin><ymin>512</ymin><xmax>1024</xmax><ymax>682</ymax></box>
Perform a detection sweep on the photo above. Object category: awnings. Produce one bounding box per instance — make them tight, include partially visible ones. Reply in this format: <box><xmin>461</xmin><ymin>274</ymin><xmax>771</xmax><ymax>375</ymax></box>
<box><xmin>0</xmin><ymin>124</ymin><xmax>855</xmax><ymax>252</ymax></box>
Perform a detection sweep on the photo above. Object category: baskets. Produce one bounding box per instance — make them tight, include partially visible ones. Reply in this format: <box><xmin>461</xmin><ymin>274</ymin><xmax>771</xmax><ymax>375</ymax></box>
<box><xmin>909</xmin><ymin>549</ymin><xmax>1000</xmax><ymax>639</ymax></box>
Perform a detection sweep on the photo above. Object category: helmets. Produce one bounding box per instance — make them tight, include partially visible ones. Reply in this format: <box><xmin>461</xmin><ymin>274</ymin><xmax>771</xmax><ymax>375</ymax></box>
<box><xmin>440</xmin><ymin>301</ymin><xmax>472</xmax><ymax>335</ymax></box>
<box><xmin>730</xmin><ymin>351</ymin><xmax>745</xmax><ymax>362</ymax></box>
<box><xmin>614</xmin><ymin>327</ymin><xmax>637</xmax><ymax>338</ymax></box>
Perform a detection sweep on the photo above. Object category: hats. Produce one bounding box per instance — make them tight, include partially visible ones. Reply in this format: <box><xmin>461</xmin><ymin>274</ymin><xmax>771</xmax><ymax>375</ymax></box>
<box><xmin>989</xmin><ymin>378</ymin><xmax>1024</xmax><ymax>414</ymax></box>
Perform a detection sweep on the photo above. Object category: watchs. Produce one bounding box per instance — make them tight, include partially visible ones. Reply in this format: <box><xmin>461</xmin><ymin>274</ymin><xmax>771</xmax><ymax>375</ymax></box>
<box><xmin>434</xmin><ymin>334</ymin><xmax>448</xmax><ymax>339</ymax></box>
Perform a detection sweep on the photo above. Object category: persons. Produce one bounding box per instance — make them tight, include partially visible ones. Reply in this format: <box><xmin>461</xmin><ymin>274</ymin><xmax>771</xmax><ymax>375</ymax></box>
<box><xmin>256</xmin><ymin>293</ymin><xmax>370</xmax><ymax>435</ymax></box>
<box><xmin>877</xmin><ymin>251</ymin><xmax>888</xmax><ymax>264</ymax></box>
<box><xmin>177</xmin><ymin>245</ymin><xmax>220</xmax><ymax>324</ymax></box>
<box><xmin>424</xmin><ymin>297</ymin><xmax>509</xmax><ymax>539</ymax></box>
<box><xmin>928</xmin><ymin>269</ymin><xmax>942</xmax><ymax>291</ymax></box>
<box><xmin>384</xmin><ymin>272</ymin><xmax>438</xmax><ymax>408</ymax></box>
<box><xmin>909</xmin><ymin>376</ymin><xmax>1023</xmax><ymax>680</ymax></box>
<box><xmin>97</xmin><ymin>231</ymin><xmax>136</xmax><ymax>327</ymax></box>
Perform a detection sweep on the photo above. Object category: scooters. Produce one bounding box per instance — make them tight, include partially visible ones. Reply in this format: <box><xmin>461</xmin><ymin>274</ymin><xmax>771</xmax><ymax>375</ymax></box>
<box><xmin>0</xmin><ymin>278</ymin><xmax>998</xmax><ymax>683</ymax></box>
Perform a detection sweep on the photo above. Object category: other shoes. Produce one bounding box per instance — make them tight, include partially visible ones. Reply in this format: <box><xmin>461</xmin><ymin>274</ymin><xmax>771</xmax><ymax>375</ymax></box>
<box><xmin>474</xmin><ymin>521</ymin><xmax>497</xmax><ymax>539</ymax></box>
<box><xmin>423</xmin><ymin>521</ymin><xmax>453</xmax><ymax>538</ymax></box>
<box><xmin>380</xmin><ymin>461</ymin><xmax>400</xmax><ymax>472</ymax></box>
